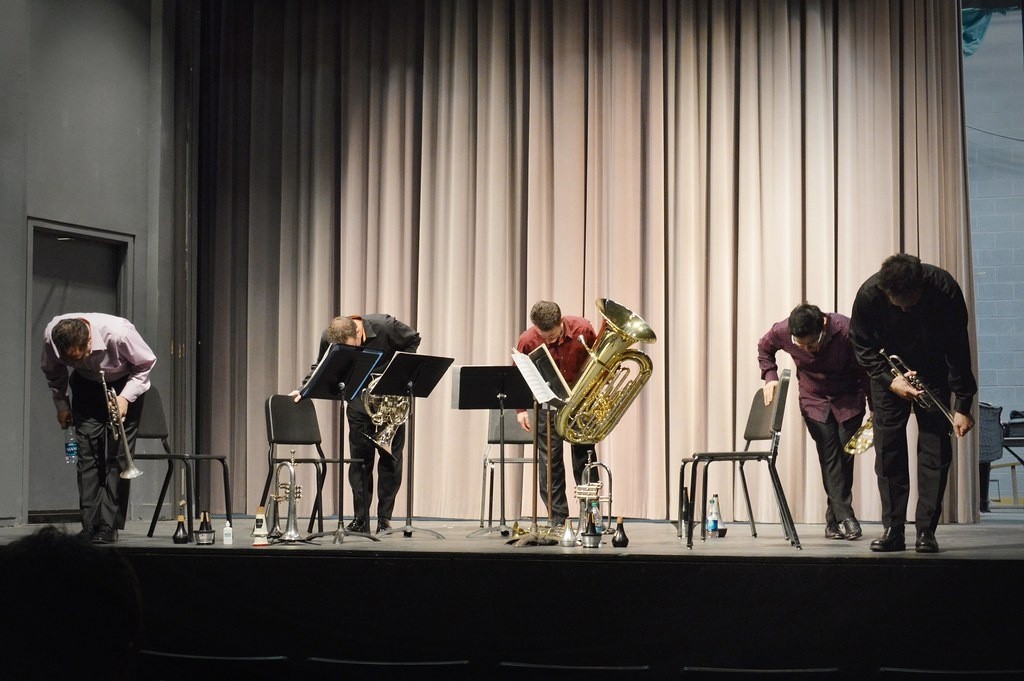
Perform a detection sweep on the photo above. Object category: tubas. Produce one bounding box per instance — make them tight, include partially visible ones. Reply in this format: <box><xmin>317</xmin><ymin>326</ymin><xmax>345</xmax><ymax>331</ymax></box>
<box><xmin>553</xmin><ymin>296</ymin><xmax>659</xmax><ymax>445</ymax></box>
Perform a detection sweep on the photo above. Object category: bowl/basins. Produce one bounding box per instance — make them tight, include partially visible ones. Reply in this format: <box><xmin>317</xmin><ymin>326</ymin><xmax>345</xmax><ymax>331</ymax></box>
<box><xmin>581</xmin><ymin>532</ymin><xmax>602</xmax><ymax>548</ymax></box>
<box><xmin>718</xmin><ymin>528</ymin><xmax>727</xmax><ymax>537</ymax></box>
<box><xmin>193</xmin><ymin>530</ymin><xmax>216</xmax><ymax>543</ymax></box>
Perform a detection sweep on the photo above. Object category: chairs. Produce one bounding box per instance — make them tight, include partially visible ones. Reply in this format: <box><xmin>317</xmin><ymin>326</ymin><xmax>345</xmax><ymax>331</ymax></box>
<box><xmin>677</xmin><ymin>369</ymin><xmax>803</xmax><ymax>549</ymax></box>
<box><xmin>480</xmin><ymin>409</ymin><xmax>539</xmax><ymax>529</ymax></box>
<box><xmin>131</xmin><ymin>647</ymin><xmax>1024</xmax><ymax>681</ymax></box>
<box><xmin>252</xmin><ymin>394</ymin><xmax>369</xmax><ymax>538</ymax></box>
<box><xmin>134</xmin><ymin>387</ymin><xmax>233</xmax><ymax>542</ymax></box>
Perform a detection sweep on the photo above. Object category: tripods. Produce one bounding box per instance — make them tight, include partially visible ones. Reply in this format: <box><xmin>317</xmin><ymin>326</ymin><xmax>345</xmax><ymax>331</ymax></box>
<box><xmin>458</xmin><ymin>346</ymin><xmax>574</xmax><ymax>547</ymax></box>
<box><xmin>369</xmin><ymin>352</ymin><xmax>455</xmax><ymax>540</ymax></box>
<box><xmin>300</xmin><ymin>342</ymin><xmax>384</xmax><ymax>543</ymax></box>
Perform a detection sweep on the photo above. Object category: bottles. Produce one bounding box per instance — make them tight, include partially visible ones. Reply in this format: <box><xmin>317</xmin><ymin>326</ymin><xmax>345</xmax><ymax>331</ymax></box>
<box><xmin>612</xmin><ymin>517</ymin><xmax>629</xmax><ymax>547</ymax></box>
<box><xmin>584</xmin><ymin>512</ymin><xmax>597</xmax><ymax>533</ymax></box>
<box><xmin>64</xmin><ymin>419</ymin><xmax>78</xmax><ymax>463</ymax></box>
<box><xmin>591</xmin><ymin>501</ymin><xmax>602</xmax><ymax>547</ymax></box>
<box><xmin>172</xmin><ymin>515</ymin><xmax>188</xmax><ymax>544</ymax></box>
<box><xmin>223</xmin><ymin>521</ymin><xmax>233</xmax><ymax>544</ymax></box>
<box><xmin>561</xmin><ymin>516</ymin><xmax>576</xmax><ymax>546</ymax></box>
<box><xmin>681</xmin><ymin>487</ymin><xmax>689</xmax><ymax>539</ymax></box>
<box><xmin>197</xmin><ymin>511</ymin><xmax>214</xmax><ymax>545</ymax></box>
<box><xmin>705</xmin><ymin>494</ymin><xmax>724</xmax><ymax>538</ymax></box>
<box><xmin>253</xmin><ymin>505</ymin><xmax>269</xmax><ymax>546</ymax></box>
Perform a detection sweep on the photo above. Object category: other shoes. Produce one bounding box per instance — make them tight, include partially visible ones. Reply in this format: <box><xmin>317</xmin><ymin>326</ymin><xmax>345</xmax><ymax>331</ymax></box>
<box><xmin>595</xmin><ymin>524</ymin><xmax>616</xmax><ymax>534</ymax></box>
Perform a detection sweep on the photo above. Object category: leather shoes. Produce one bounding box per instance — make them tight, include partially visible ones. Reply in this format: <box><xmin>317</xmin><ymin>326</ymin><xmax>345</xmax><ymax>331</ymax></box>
<box><xmin>825</xmin><ymin>518</ymin><xmax>862</xmax><ymax>540</ymax></box>
<box><xmin>914</xmin><ymin>529</ymin><xmax>939</xmax><ymax>552</ymax></box>
<box><xmin>375</xmin><ymin>518</ymin><xmax>393</xmax><ymax>535</ymax></box>
<box><xmin>346</xmin><ymin>516</ymin><xmax>370</xmax><ymax>533</ymax></box>
<box><xmin>870</xmin><ymin>526</ymin><xmax>906</xmax><ymax>551</ymax></box>
<box><xmin>76</xmin><ymin>524</ymin><xmax>118</xmax><ymax>544</ymax></box>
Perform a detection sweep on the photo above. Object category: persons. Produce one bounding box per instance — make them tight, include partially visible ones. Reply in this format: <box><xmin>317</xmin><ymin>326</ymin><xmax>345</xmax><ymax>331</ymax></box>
<box><xmin>288</xmin><ymin>313</ymin><xmax>421</xmax><ymax>535</ymax></box>
<box><xmin>849</xmin><ymin>254</ymin><xmax>977</xmax><ymax>552</ymax></box>
<box><xmin>512</xmin><ymin>300</ymin><xmax>616</xmax><ymax>534</ymax></box>
<box><xmin>756</xmin><ymin>303</ymin><xmax>874</xmax><ymax>540</ymax></box>
<box><xmin>40</xmin><ymin>312</ymin><xmax>158</xmax><ymax>544</ymax></box>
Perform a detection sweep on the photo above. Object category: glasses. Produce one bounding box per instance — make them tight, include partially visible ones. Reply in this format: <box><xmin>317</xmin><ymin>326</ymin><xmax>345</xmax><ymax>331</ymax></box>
<box><xmin>791</xmin><ymin>330</ymin><xmax>823</xmax><ymax>348</ymax></box>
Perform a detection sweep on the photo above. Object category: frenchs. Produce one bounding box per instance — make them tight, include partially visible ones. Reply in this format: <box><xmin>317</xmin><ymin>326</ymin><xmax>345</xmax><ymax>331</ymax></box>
<box><xmin>359</xmin><ymin>371</ymin><xmax>416</xmax><ymax>461</ymax></box>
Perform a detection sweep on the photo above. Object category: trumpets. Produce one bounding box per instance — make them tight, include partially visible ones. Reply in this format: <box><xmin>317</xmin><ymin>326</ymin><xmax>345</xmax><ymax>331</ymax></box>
<box><xmin>573</xmin><ymin>449</ymin><xmax>613</xmax><ymax>545</ymax></box>
<box><xmin>844</xmin><ymin>417</ymin><xmax>874</xmax><ymax>455</ymax></box>
<box><xmin>267</xmin><ymin>448</ymin><xmax>304</xmax><ymax>540</ymax></box>
<box><xmin>878</xmin><ymin>347</ymin><xmax>956</xmax><ymax>437</ymax></box>
<box><xmin>99</xmin><ymin>368</ymin><xmax>144</xmax><ymax>480</ymax></box>
